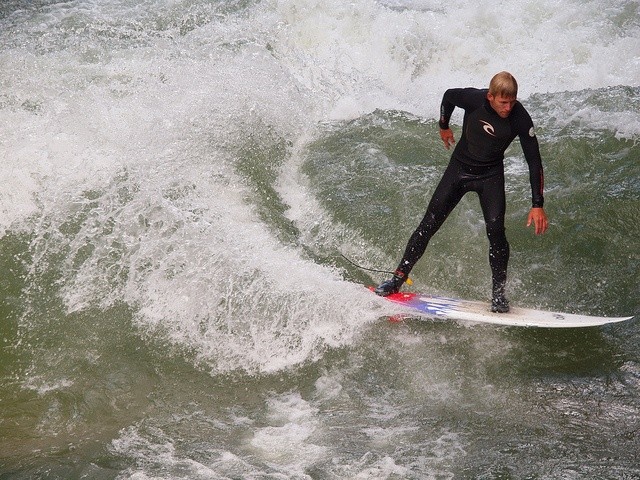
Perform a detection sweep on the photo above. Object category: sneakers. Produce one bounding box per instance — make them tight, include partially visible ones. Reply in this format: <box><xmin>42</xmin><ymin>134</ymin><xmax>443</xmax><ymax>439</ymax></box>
<box><xmin>375</xmin><ymin>277</ymin><xmax>401</xmax><ymax>296</ymax></box>
<box><xmin>492</xmin><ymin>294</ymin><xmax>510</xmax><ymax>313</ymax></box>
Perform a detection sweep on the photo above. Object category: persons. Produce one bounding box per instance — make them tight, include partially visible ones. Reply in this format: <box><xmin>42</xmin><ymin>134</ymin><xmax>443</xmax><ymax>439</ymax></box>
<box><xmin>373</xmin><ymin>71</ymin><xmax>549</xmax><ymax>314</ymax></box>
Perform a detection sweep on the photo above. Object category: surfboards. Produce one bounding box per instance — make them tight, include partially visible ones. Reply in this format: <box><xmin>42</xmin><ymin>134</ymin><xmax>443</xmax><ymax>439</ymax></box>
<box><xmin>367</xmin><ymin>285</ymin><xmax>634</xmax><ymax>328</ymax></box>
